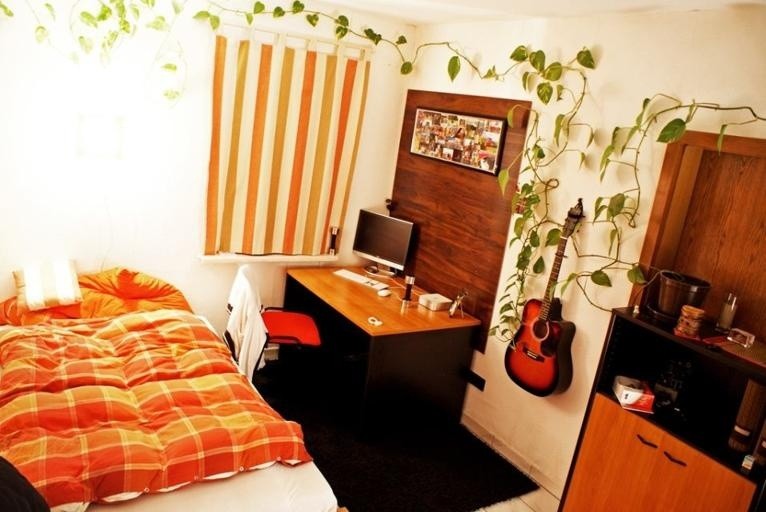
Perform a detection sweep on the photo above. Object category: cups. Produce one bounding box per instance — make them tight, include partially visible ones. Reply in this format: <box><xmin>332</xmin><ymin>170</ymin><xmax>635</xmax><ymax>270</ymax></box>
<box><xmin>677</xmin><ymin>305</ymin><xmax>707</xmax><ymax>335</ymax></box>
<box><xmin>716</xmin><ymin>291</ymin><xmax>741</xmax><ymax>334</ymax></box>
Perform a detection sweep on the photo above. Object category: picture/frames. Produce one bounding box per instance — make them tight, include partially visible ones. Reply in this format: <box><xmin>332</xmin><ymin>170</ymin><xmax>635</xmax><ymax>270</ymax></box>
<box><xmin>409</xmin><ymin>105</ymin><xmax>508</xmax><ymax>175</ymax></box>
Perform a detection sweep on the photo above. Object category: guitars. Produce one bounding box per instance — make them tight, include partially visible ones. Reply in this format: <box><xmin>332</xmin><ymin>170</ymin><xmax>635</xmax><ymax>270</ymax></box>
<box><xmin>504</xmin><ymin>200</ymin><xmax>586</xmax><ymax>399</ymax></box>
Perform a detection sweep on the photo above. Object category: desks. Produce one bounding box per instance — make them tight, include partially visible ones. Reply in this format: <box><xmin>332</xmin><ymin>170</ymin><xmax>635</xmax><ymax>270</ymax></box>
<box><xmin>279</xmin><ymin>265</ymin><xmax>481</xmax><ymax>425</ymax></box>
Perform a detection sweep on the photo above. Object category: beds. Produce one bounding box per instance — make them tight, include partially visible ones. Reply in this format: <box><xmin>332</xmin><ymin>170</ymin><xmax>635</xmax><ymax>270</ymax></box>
<box><xmin>0</xmin><ymin>268</ymin><xmax>347</xmax><ymax>511</ymax></box>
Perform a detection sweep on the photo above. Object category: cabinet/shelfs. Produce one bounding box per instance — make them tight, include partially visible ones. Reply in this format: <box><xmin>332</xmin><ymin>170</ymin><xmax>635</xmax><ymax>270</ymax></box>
<box><xmin>561</xmin><ymin>392</ymin><xmax>757</xmax><ymax>511</ymax></box>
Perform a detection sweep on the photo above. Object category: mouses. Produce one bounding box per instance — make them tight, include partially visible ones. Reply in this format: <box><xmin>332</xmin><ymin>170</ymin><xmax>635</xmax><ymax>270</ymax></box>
<box><xmin>377</xmin><ymin>290</ymin><xmax>392</xmax><ymax>297</ymax></box>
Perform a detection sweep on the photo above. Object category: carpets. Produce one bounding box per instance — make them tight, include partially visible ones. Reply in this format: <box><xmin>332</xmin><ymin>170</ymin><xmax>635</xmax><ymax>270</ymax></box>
<box><xmin>305</xmin><ymin>424</ymin><xmax>540</xmax><ymax>511</ymax></box>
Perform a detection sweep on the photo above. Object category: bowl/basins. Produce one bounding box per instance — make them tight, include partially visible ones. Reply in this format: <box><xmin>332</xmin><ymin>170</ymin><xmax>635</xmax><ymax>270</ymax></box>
<box><xmin>658</xmin><ymin>270</ymin><xmax>712</xmax><ymax>315</ymax></box>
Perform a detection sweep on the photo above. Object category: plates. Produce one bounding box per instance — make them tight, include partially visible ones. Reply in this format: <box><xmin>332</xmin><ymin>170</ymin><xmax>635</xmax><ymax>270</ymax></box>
<box><xmin>646</xmin><ymin>303</ymin><xmax>677</xmax><ymax>326</ymax></box>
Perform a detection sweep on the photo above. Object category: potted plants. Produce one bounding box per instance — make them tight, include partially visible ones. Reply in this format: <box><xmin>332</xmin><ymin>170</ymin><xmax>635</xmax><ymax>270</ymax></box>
<box><xmin>0</xmin><ymin>1</ymin><xmax>766</xmax><ymax>352</ymax></box>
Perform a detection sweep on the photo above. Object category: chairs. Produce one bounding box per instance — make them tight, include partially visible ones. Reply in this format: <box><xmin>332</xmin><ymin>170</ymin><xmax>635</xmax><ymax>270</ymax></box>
<box><xmin>222</xmin><ymin>264</ymin><xmax>322</xmax><ymax>355</ymax></box>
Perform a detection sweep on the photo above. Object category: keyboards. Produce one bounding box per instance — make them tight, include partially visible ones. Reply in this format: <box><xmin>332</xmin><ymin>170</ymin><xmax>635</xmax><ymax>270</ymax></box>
<box><xmin>333</xmin><ymin>269</ymin><xmax>390</xmax><ymax>291</ymax></box>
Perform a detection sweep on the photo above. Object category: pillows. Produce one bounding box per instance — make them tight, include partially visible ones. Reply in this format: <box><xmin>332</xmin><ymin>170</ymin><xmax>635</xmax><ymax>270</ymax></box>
<box><xmin>14</xmin><ymin>257</ymin><xmax>83</xmax><ymax>317</ymax></box>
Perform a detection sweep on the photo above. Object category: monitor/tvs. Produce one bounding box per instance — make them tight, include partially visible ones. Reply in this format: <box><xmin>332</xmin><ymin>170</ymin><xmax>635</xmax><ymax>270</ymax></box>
<box><xmin>352</xmin><ymin>209</ymin><xmax>414</xmax><ymax>278</ymax></box>
